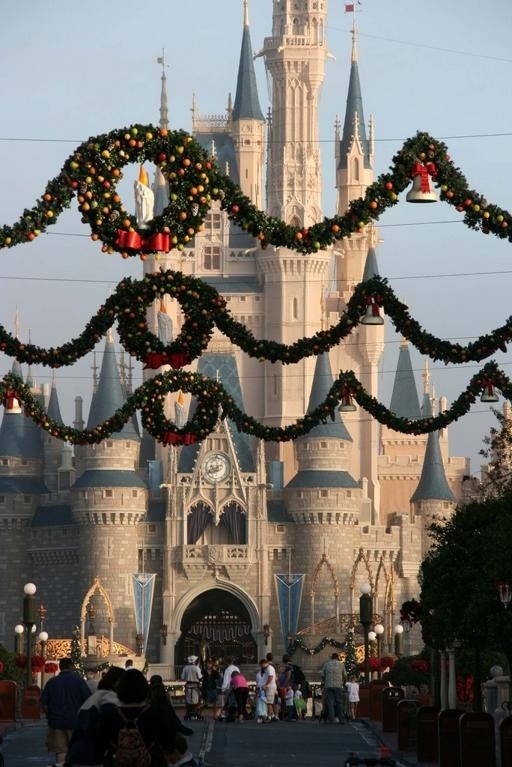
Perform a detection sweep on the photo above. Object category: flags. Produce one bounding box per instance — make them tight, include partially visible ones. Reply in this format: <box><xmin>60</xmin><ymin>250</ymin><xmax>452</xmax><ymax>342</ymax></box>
<box><xmin>345</xmin><ymin>3</ymin><xmax>354</xmax><ymax>12</ymax></box>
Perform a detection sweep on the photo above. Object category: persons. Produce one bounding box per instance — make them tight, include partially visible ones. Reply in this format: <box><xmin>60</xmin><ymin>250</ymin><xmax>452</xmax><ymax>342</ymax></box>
<box><xmin>179</xmin><ymin>653</ymin><xmax>361</xmax><ymax>725</ymax></box>
<box><xmin>39</xmin><ymin>655</ymin><xmax>94</xmax><ymax>766</ymax></box>
<box><xmin>63</xmin><ymin>658</ymin><xmax>198</xmax><ymax>766</ymax></box>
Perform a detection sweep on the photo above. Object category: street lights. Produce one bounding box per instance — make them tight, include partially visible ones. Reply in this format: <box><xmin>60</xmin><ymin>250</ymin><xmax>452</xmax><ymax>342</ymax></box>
<box><xmin>369</xmin><ymin>624</ymin><xmax>384</xmax><ymax>681</ymax></box>
<box><xmin>14</xmin><ymin>582</ymin><xmax>49</xmax><ymax>689</ymax></box>
<box><xmin>360</xmin><ymin>582</ymin><xmax>372</xmax><ymax>686</ymax></box>
<box><xmin>394</xmin><ymin>624</ymin><xmax>404</xmax><ymax>660</ymax></box>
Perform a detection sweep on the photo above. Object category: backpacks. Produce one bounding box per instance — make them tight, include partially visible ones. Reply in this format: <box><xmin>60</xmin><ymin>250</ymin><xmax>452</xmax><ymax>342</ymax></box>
<box><xmin>116</xmin><ymin>705</ymin><xmax>152</xmax><ymax>767</ymax></box>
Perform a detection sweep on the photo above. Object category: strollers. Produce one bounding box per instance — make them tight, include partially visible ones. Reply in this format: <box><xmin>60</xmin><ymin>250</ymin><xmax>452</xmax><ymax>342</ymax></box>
<box><xmin>216</xmin><ymin>687</ymin><xmax>237</xmax><ymax>722</ymax></box>
<box><xmin>184</xmin><ymin>682</ymin><xmax>208</xmax><ymax>720</ymax></box>
